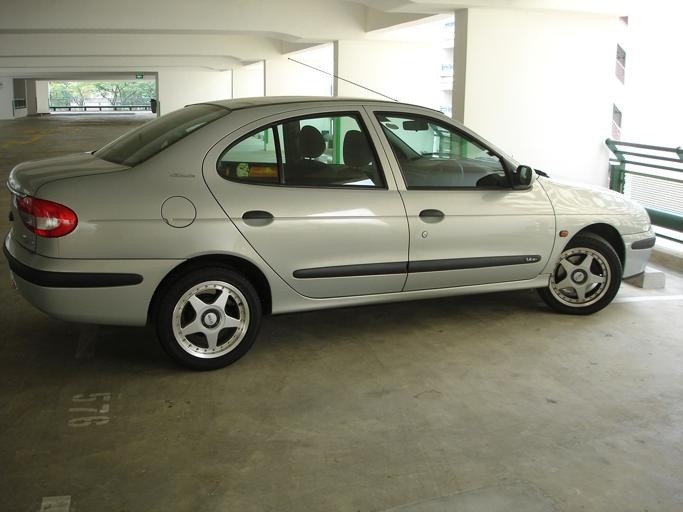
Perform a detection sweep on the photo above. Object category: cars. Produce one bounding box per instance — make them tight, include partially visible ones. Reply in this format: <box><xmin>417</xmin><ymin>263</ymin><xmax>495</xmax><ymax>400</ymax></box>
<box><xmin>1</xmin><ymin>95</ymin><xmax>656</xmax><ymax>372</ymax></box>
